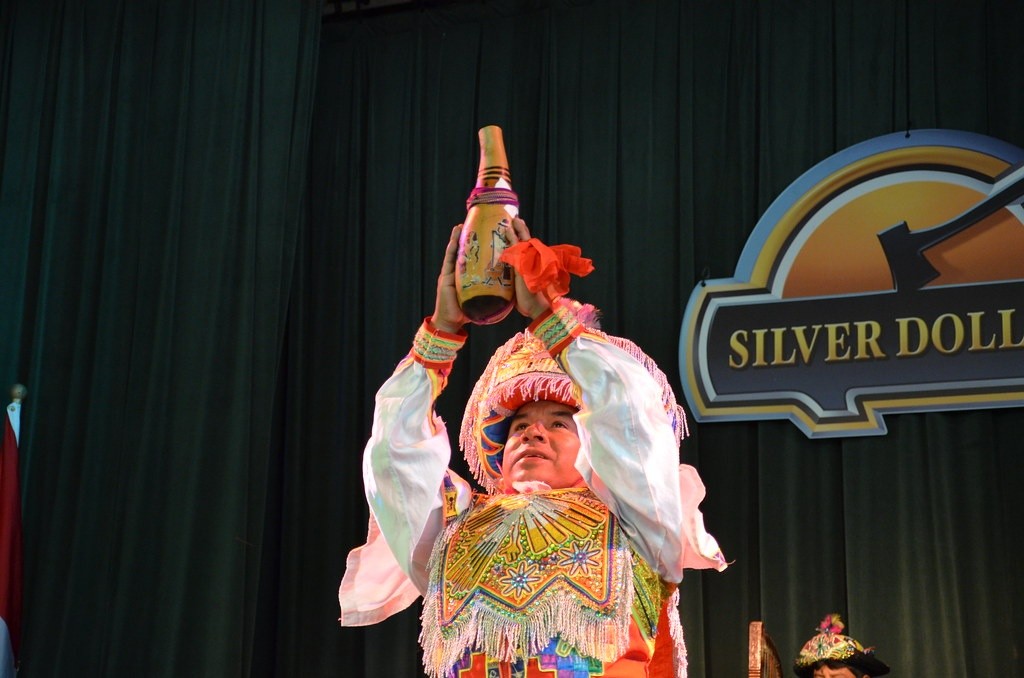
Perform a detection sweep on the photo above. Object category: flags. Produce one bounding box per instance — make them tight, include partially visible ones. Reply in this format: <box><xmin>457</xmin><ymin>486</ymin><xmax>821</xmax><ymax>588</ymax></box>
<box><xmin>0</xmin><ymin>399</ymin><xmax>24</xmax><ymax>678</ymax></box>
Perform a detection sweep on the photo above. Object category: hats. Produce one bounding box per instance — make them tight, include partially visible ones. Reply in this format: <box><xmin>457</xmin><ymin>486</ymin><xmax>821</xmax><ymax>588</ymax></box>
<box><xmin>792</xmin><ymin>615</ymin><xmax>890</xmax><ymax>678</ymax></box>
<box><xmin>459</xmin><ymin>297</ymin><xmax>689</xmax><ymax>498</ymax></box>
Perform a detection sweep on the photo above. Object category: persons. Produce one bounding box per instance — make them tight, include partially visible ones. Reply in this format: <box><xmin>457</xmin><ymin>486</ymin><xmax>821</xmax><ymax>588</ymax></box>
<box><xmin>794</xmin><ymin>615</ymin><xmax>889</xmax><ymax>678</ymax></box>
<box><xmin>339</xmin><ymin>221</ymin><xmax>727</xmax><ymax>677</ymax></box>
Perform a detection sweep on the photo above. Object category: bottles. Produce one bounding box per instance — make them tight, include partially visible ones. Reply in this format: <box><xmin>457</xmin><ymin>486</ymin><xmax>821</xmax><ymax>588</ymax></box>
<box><xmin>455</xmin><ymin>125</ymin><xmax>518</xmax><ymax>326</ymax></box>
